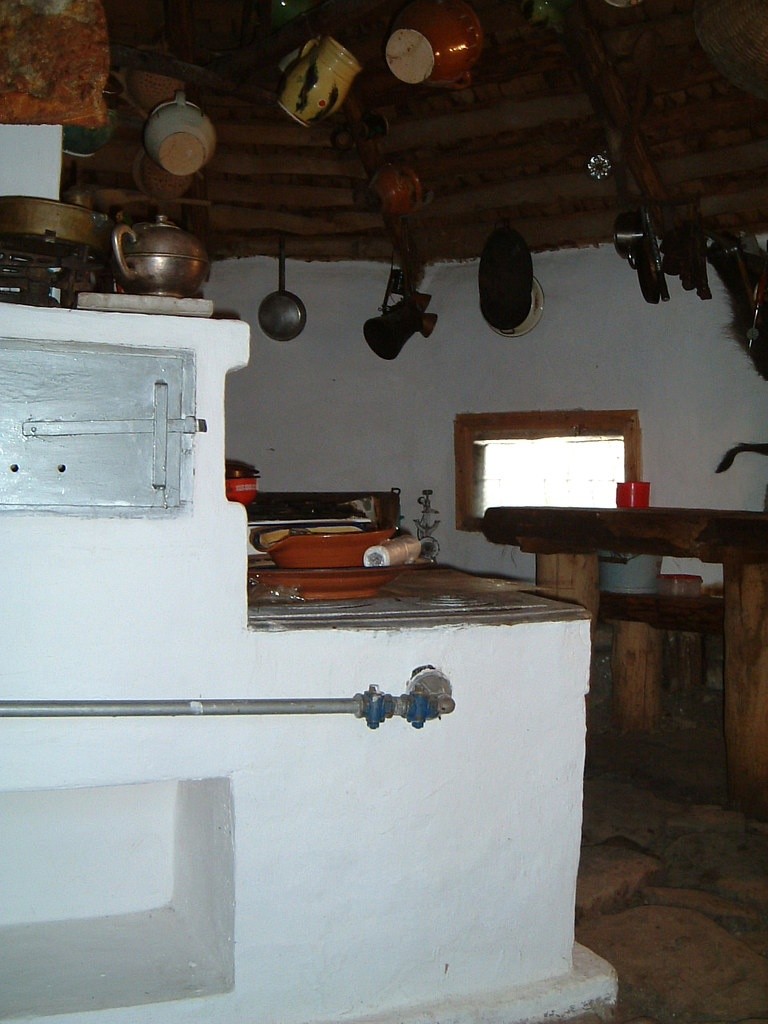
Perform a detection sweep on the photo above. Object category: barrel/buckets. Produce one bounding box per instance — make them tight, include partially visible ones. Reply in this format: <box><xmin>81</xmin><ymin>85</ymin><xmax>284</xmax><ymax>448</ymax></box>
<box><xmin>598</xmin><ymin>551</ymin><xmax>662</xmax><ymax>594</ymax></box>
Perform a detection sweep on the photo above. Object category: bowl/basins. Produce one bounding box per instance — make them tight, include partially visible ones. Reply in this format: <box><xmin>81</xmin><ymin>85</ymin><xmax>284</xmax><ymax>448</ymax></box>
<box><xmin>248</xmin><ymin>554</ymin><xmax>400</xmax><ymax>601</ymax></box>
<box><xmin>250</xmin><ymin>527</ymin><xmax>397</xmax><ymax>568</ymax></box>
<box><xmin>658</xmin><ymin>574</ymin><xmax>703</xmax><ymax>595</ymax></box>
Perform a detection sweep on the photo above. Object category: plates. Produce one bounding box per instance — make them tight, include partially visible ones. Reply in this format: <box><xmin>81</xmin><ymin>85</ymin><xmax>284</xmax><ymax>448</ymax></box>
<box><xmin>487</xmin><ymin>277</ymin><xmax>544</xmax><ymax>338</ymax></box>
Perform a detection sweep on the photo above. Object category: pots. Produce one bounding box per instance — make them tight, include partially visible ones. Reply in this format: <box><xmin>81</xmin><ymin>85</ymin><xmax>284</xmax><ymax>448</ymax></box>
<box><xmin>479</xmin><ymin>221</ymin><xmax>534</xmax><ymax>334</ymax></box>
<box><xmin>614</xmin><ymin>207</ymin><xmax>643</xmax><ymax>269</ymax></box>
<box><xmin>258</xmin><ymin>236</ymin><xmax>307</xmax><ymax>342</ymax></box>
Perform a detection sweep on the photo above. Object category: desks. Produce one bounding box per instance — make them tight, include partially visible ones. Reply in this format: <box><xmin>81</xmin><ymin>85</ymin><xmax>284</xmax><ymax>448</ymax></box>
<box><xmin>481</xmin><ymin>507</ymin><xmax>768</xmax><ymax>817</ymax></box>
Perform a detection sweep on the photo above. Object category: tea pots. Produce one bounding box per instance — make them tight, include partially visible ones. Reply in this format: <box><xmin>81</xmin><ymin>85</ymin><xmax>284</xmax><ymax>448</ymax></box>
<box><xmin>111</xmin><ymin>214</ymin><xmax>212</xmax><ymax>299</ymax></box>
<box><xmin>144</xmin><ymin>92</ymin><xmax>217</xmax><ymax>177</ymax></box>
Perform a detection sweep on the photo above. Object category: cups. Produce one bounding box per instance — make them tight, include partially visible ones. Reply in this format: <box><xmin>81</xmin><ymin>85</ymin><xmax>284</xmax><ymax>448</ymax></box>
<box><xmin>617</xmin><ymin>482</ymin><xmax>650</xmax><ymax>509</ymax></box>
